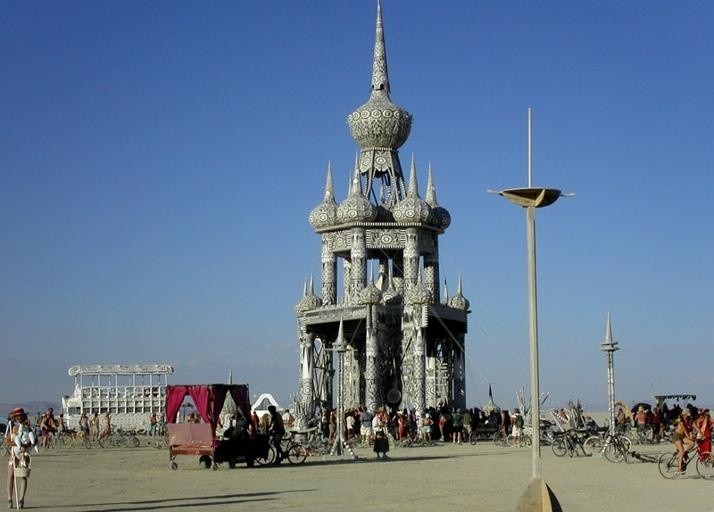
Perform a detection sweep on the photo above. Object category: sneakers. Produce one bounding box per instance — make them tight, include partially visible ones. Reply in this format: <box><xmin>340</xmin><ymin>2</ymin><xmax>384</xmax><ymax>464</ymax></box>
<box><xmin>7</xmin><ymin>499</ymin><xmax>24</xmax><ymax>509</ymax></box>
<box><xmin>678</xmin><ymin>469</ymin><xmax>686</xmax><ymax>474</ymax></box>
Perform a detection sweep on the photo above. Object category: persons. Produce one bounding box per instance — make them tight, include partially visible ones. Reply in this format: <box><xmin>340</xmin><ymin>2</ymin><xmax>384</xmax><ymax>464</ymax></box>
<box><xmin>615</xmin><ymin>403</ymin><xmax>699</xmax><ymax>444</ymax></box>
<box><xmin>672</xmin><ymin>408</ymin><xmax>706</xmax><ymax>476</ymax></box>
<box><xmin>226</xmin><ymin>406</ymin><xmax>296</xmax><ymax>464</ymax></box>
<box><xmin>4</xmin><ymin>407</ymin><xmax>39</xmax><ymax>509</ymax></box>
<box><xmin>304</xmin><ymin>401</ymin><xmax>523</xmax><ymax>461</ymax></box>
<box><xmin>1</xmin><ymin>402</ymin><xmax>170</xmax><ymax>450</ymax></box>
<box><xmin>554</xmin><ymin>408</ymin><xmax>598</xmax><ymax>432</ymax></box>
<box><xmin>180</xmin><ymin>399</ymin><xmax>714</xmax><ymax>464</ymax></box>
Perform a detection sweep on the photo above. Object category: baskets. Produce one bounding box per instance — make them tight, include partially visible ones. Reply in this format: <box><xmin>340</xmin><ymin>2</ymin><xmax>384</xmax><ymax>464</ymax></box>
<box><xmin>15</xmin><ymin>453</ymin><xmax>32</xmax><ymax>477</ymax></box>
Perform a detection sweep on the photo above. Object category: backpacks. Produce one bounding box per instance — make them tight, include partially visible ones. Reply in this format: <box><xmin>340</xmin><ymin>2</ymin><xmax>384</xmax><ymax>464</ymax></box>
<box><xmin>515</xmin><ymin>415</ymin><xmax>524</xmax><ymax>428</ymax></box>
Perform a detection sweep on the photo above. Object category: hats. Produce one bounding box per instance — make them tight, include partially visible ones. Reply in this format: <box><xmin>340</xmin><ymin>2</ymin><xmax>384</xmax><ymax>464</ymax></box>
<box><xmin>10</xmin><ymin>407</ymin><xmax>29</xmax><ymax>417</ymax></box>
<box><xmin>682</xmin><ymin>409</ymin><xmax>691</xmax><ymax>416</ymax></box>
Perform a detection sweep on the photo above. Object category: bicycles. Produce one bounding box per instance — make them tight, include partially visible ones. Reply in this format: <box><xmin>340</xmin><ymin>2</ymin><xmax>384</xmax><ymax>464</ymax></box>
<box><xmin>256</xmin><ymin>430</ymin><xmax>307</xmax><ymax>465</ymax></box>
<box><xmin>551</xmin><ymin>426</ymin><xmax>660</xmax><ymax>464</ymax></box>
<box><xmin>507</xmin><ymin>426</ymin><xmax>532</xmax><ymax>448</ymax></box>
<box><xmin>36</xmin><ymin>429</ymin><xmax>139</xmax><ymax>455</ymax></box>
<box><xmin>658</xmin><ymin>438</ymin><xmax>713</xmax><ymax>480</ymax></box>
